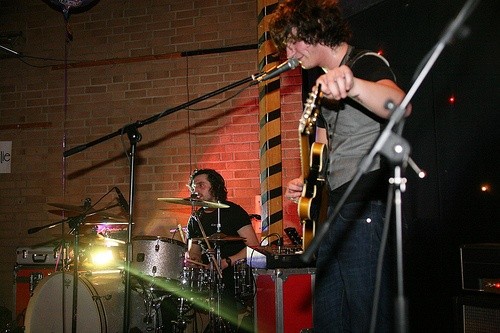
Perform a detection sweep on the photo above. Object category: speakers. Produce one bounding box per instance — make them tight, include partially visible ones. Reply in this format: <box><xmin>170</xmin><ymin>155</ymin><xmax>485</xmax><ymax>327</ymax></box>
<box><xmin>451</xmin><ymin>293</ymin><xmax>500</xmax><ymax>333</ymax></box>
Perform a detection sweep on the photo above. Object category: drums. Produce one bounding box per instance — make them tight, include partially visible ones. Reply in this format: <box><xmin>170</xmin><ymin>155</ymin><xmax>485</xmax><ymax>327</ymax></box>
<box><xmin>124</xmin><ymin>235</ymin><xmax>189</xmax><ymax>294</ymax></box>
<box><xmin>181</xmin><ymin>267</ymin><xmax>224</xmax><ymax>314</ymax></box>
<box><xmin>24</xmin><ymin>269</ymin><xmax>163</xmax><ymax>333</ymax></box>
<box><xmin>233</xmin><ymin>254</ymin><xmax>288</xmax><ymax>301</ymax></box>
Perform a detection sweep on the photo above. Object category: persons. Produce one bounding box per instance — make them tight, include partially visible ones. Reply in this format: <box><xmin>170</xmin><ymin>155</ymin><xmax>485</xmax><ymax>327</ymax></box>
<box><xmin>156</xmin><ymin>169</ymin><xmax>260</xmax><ymax>333</ymax></box>
<box><xmin>271</xmin><ymin>0</ymin><xmax>411</xmax><ymax>333</ymax></box>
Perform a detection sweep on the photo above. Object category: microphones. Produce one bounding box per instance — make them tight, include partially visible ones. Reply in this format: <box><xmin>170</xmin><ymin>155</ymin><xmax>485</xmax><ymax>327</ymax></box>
<box><xmin>116</xmin><ymin>189</ymin><xmax>129</xmax><ymax>212</ymax></box>
<box><xmin>250</xmin><ymin>57</ymin><xmax>299</xmax><ymax>85</ymax></box>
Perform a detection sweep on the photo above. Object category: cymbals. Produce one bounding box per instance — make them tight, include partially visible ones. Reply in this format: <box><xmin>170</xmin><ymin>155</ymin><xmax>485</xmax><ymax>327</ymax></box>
<box><xmin>157</xmin><ymin>196</ymin><xmax>230</xmax><ymax>209</ymax></box>
<box><xmin>191</xmin><ymin>233</ymin><xmax>248</xmax><ymax>242</ymax></box>
<box><xmin>46</xmin><ymin>201</ymin><xmax>109</xmax><ymax>217</ymax></box>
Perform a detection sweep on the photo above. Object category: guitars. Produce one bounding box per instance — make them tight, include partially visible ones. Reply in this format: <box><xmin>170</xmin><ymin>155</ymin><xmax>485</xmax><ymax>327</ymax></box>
<box><xmin>296</xmin><ymin>83</ymin><xmax>326</xmax><ymax>263</ymax></box>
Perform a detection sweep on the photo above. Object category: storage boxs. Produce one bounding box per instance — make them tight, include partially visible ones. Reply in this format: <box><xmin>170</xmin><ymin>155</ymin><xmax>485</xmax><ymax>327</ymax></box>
<box><xmin>11</xmin><ymin>264</ymin><xmax>65</xmax><ymax>323</ymax></box>
<box><xmin>251</xmin><ymin>266</ymin><xmax>318</xmax><ymax>332</ymax></box>
